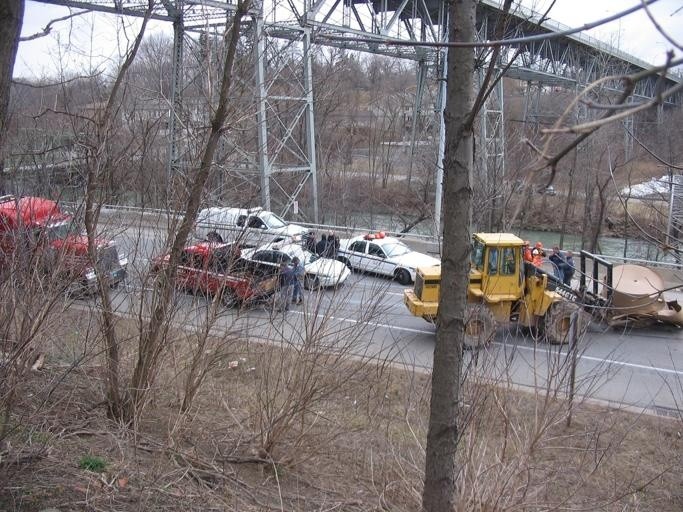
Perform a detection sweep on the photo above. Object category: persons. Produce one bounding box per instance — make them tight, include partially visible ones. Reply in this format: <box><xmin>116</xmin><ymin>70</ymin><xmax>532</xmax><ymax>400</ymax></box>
<box><xmin>291</xmin><ymin>256</ymin><xmax>305</xmax><ymax>305</ymax></box>
<box><xmin>315</xmin><ymin>232</ymin><xmax>332</xmax><ymax>258</ymax></box>
<box><xmin>530</xmin><ymin>242</ymin><xmax>546</xmax><ymax>267</ymax></box>
<box><xmin>328</xmin><ymin>230</ymin><xmax>339</xmax><ymax>253</ymax></box>
<box><xmin>562</xmin><ymin>249</ymin><xmax>575</xmax><ymax>287</ymax></box>
<box><xmin>522</xmin><ymin>240</ymin><xmax>532</xmax><ymax>262</ymax></box>
<box><xmin>276</xmin><ymin>262</ymin><xmax>295</xmax><ymax>313</ymax></box>
<box><xmin>305</xmin><ymin>231</ymin><xmax>317</xmax><ymax>252</ymax></box>
<box><xmin>548</xmin><ymin>246</ymin><xmax>565</xmax><ymax>279</ymax></box>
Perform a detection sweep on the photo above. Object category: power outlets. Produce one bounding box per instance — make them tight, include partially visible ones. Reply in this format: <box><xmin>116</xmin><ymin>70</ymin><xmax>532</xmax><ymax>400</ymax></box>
<box><xmin>147</xmin><ymin>241</ymin><xmax>287</xmax><ymax>310</ymax></box>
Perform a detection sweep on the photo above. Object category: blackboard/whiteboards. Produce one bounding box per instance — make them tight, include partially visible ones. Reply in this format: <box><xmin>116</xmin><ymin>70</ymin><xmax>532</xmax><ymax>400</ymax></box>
<box><xmin>402</xmin><ymin>230</ymin><xmax>617</xmax><ymax>351</ymax></box>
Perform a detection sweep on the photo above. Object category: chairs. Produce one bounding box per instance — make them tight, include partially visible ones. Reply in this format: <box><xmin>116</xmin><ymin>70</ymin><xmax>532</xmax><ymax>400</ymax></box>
<box><xmin>292</xmin><ymin>300</ymin><xmax>303</xmax><ymax>305</ymax></box>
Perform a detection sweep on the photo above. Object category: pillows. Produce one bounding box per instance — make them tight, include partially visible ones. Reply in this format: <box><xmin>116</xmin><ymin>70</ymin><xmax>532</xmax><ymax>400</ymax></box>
<box><xmin>365</xmin><ymin>231</ymin><xmax>385</xmax><ymax>239</ymax></box>
<box><xmin>524</xmin><ymin>240</ymin><xmax>543</xmax><ymax>254</ymax></box>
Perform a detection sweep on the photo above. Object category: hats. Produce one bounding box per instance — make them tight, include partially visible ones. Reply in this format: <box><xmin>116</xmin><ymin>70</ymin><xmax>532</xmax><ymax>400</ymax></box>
<box><xmin>334</xmin><ymin>230</ymin><xmax>441</xmax><ymax>286</ymax></box>
<box><xmin>238</xmin><ymin>236</ymin><xmax>352</xmax><ymax>291</ymax></box>
<box><xmin>543</xmin><ymin>185</ymin><xmax>556</xmax><ymax>196</ymax></box>
